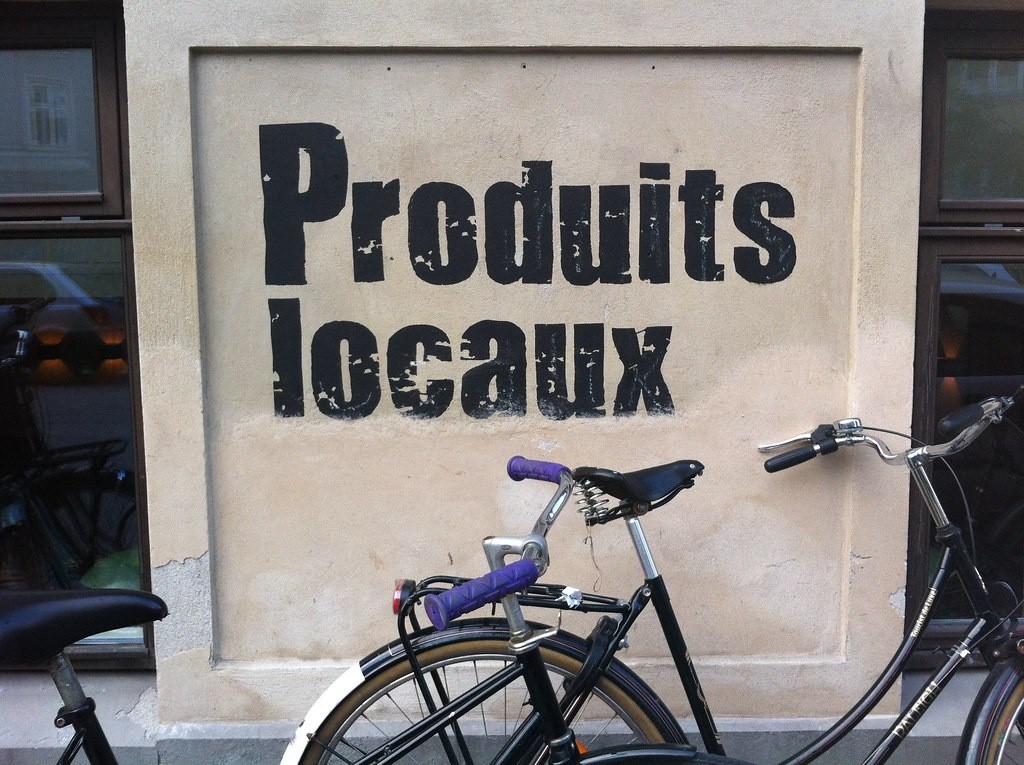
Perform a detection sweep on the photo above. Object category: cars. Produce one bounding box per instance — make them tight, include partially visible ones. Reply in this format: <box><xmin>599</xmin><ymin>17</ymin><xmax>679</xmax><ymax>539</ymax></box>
<box><xmin>1</xmin><ymin>262</ymin><xmax>112</xmax><ymax>334</ymax></box>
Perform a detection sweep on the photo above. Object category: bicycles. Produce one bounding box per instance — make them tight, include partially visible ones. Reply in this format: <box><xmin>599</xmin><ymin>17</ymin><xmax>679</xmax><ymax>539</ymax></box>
<box><xmin>279</xmin><ymin>387</ymin><xmax>1023</xmax><ymax>765</ymax></box>
<box><xmin>0</xmin><ymin>456</ymin><xmax>758</xmax><ymax>765</ymax></box>
<box><xmin>0</xmin><ymin>273</ymin><xmax>139</xmax><ymax>590</ymax></box>
